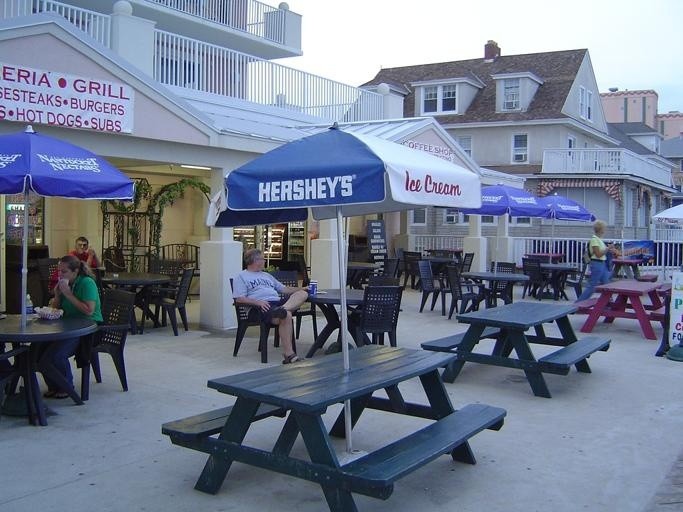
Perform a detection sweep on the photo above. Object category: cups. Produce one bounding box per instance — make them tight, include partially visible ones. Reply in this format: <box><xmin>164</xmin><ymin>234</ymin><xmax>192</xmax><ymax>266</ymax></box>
<box><xmin>309</xmin><ymin>283</ymin><xmax>317</xmax><ymax>295</ymax></box>
<box><xmin>310</xmin><ymin>280</ymin><xmax>317</xmax><ymax>292</ymax></box>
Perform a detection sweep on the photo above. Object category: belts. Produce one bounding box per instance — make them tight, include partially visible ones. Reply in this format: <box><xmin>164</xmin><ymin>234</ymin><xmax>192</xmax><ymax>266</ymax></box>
<box><xmin>591</xmin><ymin>259</ymin><xmax>607</xmax><ymax>263</ymax></box>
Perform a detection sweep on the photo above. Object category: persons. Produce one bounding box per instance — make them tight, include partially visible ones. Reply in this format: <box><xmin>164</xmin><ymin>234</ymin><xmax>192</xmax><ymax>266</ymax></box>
<box><xmin>49</xmin><ymin>236</ymin><xmax>99</xmax><ymax>294</ymax></box>
<box><xmin>573</xmin><ymin>219</ymin><xmax>617</xmax><ymax>304</ymax></box>
<box><xmin>232</xmin><ymin>247</ymin><xmax>309</xmax><ymax>365</ymax></box>
<box><xmin>42</xmin><ymin>253</ymin><xmax>105</xmax><ymax>399</ymax></box>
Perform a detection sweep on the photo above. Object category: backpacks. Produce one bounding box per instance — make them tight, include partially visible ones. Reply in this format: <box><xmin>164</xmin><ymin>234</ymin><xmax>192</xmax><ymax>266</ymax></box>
<box><xmin>582</xmin><ymin>251</ymin><xmax>595</xmax><ymax>264</ymax></box>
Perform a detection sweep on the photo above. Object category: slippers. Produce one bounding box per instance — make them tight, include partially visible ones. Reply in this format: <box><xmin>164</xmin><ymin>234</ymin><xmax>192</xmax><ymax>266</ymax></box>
<box><xmin>44</xmin><ymin>387</ymin><xmax>60</xmax><ymax>397</ymax></box>
<box><xmin>52</xmin><ymin>385</ymin><xmax>74</xmax><ymax>399</ymax></box>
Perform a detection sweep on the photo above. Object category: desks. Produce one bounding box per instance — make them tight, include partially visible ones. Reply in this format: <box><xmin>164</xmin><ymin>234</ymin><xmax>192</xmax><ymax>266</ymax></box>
<box><xmin>441</xmin><ymin>301</ymin><xmax>591</xmax><ymax>398</ymax></box>
<box><xmin>194</xmin><ymin>345</ymin><xmax>477</xmax><ymax>512</ymax></box>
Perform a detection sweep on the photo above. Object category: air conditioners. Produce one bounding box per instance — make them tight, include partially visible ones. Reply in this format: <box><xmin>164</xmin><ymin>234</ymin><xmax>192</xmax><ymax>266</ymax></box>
<box><xmin>512</xmin><ymin>153</ymin><xmax>525</xmax><ymax>162</ymax></box>
<box><xmin>445</xmin><ymin>215</ymin><xmax>458</xmax><ymax>223</ymax></box>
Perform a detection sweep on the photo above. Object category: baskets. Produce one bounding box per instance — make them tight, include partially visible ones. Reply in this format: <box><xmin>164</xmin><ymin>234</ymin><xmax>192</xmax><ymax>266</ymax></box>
<box><xmin>40</xmin><ymin>311</ymin><xmax>61</xmax><ymax>320</ymax></box>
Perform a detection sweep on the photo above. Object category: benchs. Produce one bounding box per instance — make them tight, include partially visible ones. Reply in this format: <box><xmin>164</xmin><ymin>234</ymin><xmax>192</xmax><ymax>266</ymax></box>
<box><xmin>338</xmin><ymin>402</ymin><xmax>506</xmax><ymax>487</ymax></box>
<box><xmin>162</xmin><ymin>401</ymin><xmax>286</xmax><ymax>441</ymax></box>
<box><xmin>539</xmin><ymin>336</ymin><xmax>612</xmax><ymax>367</ymax></box>
<box><xmin>570</xmin><ymin>274</ymin><xmax>672</xmax><ymax>321</ymax></box>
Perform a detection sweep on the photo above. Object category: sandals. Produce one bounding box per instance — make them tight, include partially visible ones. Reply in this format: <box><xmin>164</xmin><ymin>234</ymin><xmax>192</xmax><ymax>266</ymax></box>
<box><xmin>269</xmin><ymin>306</ymin><xmax>287</xmax><ymax>319</ymax></box>
<box><xmin>282</xmin><ymin>353</ymin><xmax>308</xmax><ymax>364</ymax></box>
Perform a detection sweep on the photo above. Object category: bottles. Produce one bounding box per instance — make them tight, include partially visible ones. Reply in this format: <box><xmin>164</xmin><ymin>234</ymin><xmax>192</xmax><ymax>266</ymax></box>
<box><xmin>290</xmin><ymin>237</ymin><xmax>304</xmax><ymax>244</ymax></box>
<box><xmin>291</xmin><ymin>229</ymin><xmax>304</xmax><ymax>236</ymax></box>
<box><xmin>26</xmin><ymin>294</ymin><xmax>33</xmax><ymax>321</ymax></box>
<box><xmin>289</xmin><ymin>246</ymin><xmax>303</xmax><ymax>253</ymax></box>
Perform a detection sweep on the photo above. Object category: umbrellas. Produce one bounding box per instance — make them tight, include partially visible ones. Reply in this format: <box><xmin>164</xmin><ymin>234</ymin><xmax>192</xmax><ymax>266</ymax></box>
<box><xmin>541</xmin><ymin>191</ymin><xmax>596</xmax><ymax>278</ymax></box>
<box><xmin>651</xmin><ymin>201</ymin><xmax>683</xmax><ymax>231</ymax></box>
<box><xmin>457</xmin><ymin>181</ymin><xmax>552</xmax><ymax>290</ymax></box>
<box><xmin>0</xmin><ymin>122</ymin><xmax>140</xmax><ymax>333</ymax></box>
<box><xmin>206</xmin><ymin>120</ymin><xmax>483</xmax><ymax>455</ymax></box>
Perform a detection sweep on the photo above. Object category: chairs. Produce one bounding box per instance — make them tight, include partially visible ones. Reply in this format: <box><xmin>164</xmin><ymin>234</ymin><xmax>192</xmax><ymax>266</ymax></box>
<box><xmin>1</xmin><ymin>257</ymin><xmax>197</xmax><ymax>426</ymax></box>
